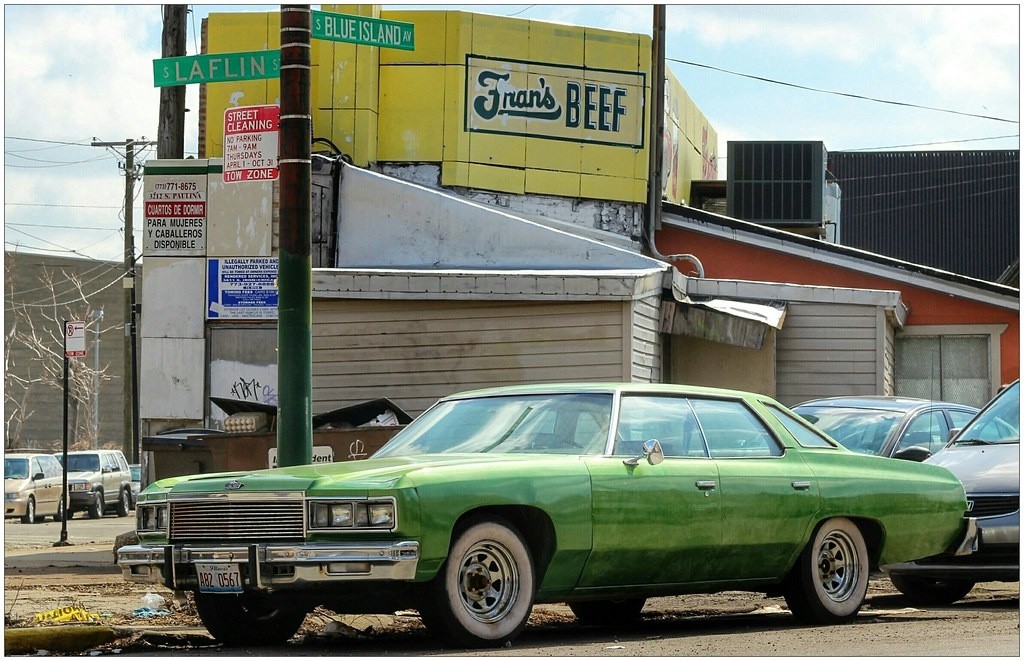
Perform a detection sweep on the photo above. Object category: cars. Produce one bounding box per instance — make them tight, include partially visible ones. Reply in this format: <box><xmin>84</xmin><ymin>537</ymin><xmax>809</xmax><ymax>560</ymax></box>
<box><xmin>118</xmin><ymin>379</ymin><xmax>1020</xmax><ymax>647</ymax></box>
<box><xmin>129</xmin><ymin>464</ymin><xmax>141</xmax><ymax>510</ymax></box>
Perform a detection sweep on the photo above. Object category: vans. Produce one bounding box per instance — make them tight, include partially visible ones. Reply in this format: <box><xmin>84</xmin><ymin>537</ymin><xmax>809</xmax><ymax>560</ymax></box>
<box><xmin>4</xmin><ymin>450</ymin><xmax>132</xmax><ymax>524</ymax></box>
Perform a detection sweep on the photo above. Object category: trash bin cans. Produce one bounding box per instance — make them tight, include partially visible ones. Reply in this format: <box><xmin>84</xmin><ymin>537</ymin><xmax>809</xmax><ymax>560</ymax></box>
<box><xmin>144</xmin><ymin>396</ymin><xmax>418</xmax><ymax>491</ymax></box>
